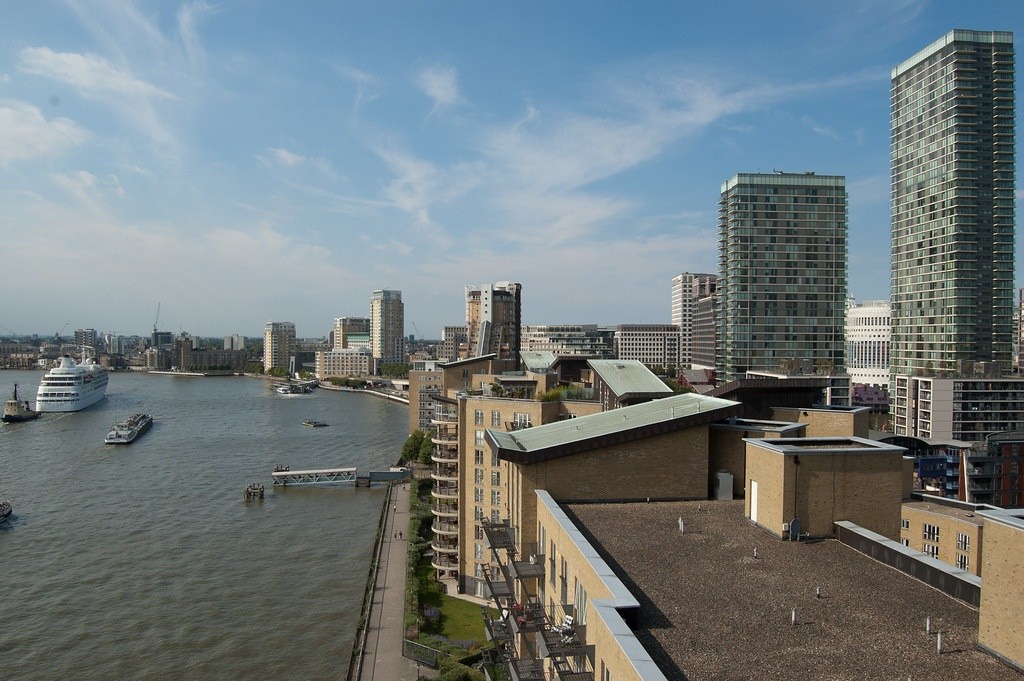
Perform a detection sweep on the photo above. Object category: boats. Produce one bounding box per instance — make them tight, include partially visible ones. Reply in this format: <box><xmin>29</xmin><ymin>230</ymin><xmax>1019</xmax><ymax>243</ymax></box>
<box><xmin>1</xmin><ymin>384</ymin><xmax>40</xmax><ymax>422</ymax></box>
<box><xmin>104</xmin><ymin>412</ymin><xmax>154</xmax><ymax>444</ymax></box>
<box><xmin>275</xmin><ymin>384</ymin><xmax>312</xmax><ymax>394</ymax></box>
<box><xmin>0</xmin><ymin>498</ymin><xmax>13</xmax><ymax>522</ymax></box>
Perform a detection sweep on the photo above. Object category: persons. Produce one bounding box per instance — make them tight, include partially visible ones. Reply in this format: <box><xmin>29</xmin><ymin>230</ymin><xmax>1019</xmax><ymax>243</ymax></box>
<box><xmin>393</xmin><ymin>530</ymin><xmax>397</xmax><ymax>540</ymax></box>
<box><xmin>402</xmin><ymin>484</ymin><xmax>406</xmax><ymax>491</ymax></box>
<box><xmin>398</xmin><ymin>530</ymin><xmax>402</xmax><ymax>541</ymax></box>
<box><xmin>393</xmin><ymin>504</ymin><xmax>396</xmax><ymax>513</ymax></box>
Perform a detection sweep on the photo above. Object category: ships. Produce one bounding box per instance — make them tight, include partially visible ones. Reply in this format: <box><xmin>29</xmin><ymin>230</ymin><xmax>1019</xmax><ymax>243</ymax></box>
<box><xmin>35</xmin><ymin>344</ymin><xmax>110</xmax><ymax>413</ymax></box>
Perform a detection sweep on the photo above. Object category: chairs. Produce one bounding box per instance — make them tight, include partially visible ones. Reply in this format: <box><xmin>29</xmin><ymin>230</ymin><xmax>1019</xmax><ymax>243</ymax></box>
<box><xmin>553</xmin><ymin>614</ymin><xmax>577</xmax><ymax>645</ymax></box>
